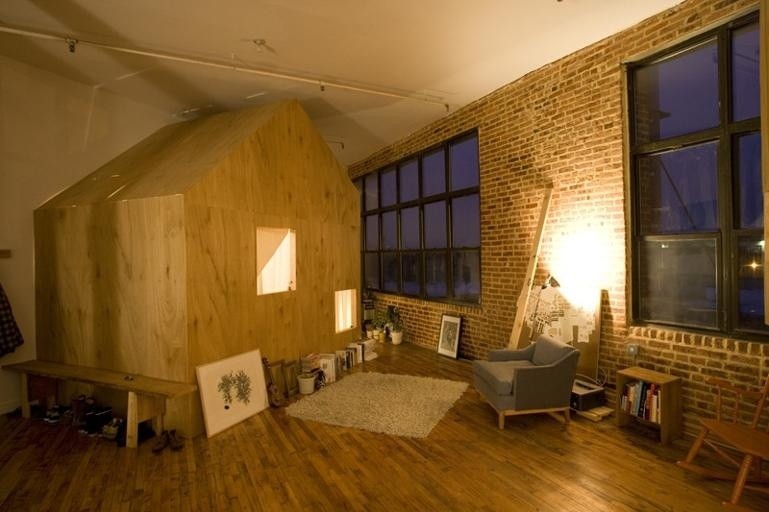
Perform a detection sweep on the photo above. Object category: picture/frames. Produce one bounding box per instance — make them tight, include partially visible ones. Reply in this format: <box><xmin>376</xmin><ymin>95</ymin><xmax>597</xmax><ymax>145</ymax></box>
<box><xmin>265</xmin><ymin>359</ymin><xmax>300</xmax><ymax>397</ymax></box>
<box><xmin>196</xmin><ymin>348</ymin><xmax>270</xmax><ymax>438</ymax></box>
<box><xmin>437</xmin><ymin>314</ymin><xmax>463</xmax><ymax>359</ymax></box>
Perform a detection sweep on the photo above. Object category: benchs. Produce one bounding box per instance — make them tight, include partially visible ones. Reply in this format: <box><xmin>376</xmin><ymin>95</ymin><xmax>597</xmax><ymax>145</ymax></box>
<box><xmin>2</xmin><ymin>359</ymin><xmax>198</xmax><ymax>449</ymax></box>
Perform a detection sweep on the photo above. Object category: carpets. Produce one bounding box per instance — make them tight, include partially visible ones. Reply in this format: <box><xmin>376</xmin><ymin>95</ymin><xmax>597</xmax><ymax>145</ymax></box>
<box><xmin>285</xmin><ymin>371</ymin><xmax>472</xmax><ymax>438</ymax></box>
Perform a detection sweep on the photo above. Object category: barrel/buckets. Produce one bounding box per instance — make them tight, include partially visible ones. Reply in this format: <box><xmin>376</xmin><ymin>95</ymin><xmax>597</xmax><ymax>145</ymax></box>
<box><xmin>297</xmin><ymin>373</ymin><xmax>316</xmax><ymax>395</ymax></box>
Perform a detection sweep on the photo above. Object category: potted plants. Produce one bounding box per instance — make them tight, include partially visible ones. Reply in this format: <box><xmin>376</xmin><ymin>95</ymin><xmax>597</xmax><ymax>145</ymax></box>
<box><xmin>366</xmin><ymin>304</ymin><xmax>405</xmax><ymax>345</ymax></box>
<box><xmin>296</xmin><ymin>368</ymin><xmax>322</xmax><ymax>395</ymax></box>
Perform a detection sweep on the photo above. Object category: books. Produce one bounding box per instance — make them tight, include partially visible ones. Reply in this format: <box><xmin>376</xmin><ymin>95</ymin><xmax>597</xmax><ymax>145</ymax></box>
<box><xmin>320</xmin><ymin>342</ymin><xmax>364</xmax><ymax>384</ymax></box>
<box><xmin>619</xmin><ymin>380</ymin><xmax>662</xmax><ymax>425</ymax></box>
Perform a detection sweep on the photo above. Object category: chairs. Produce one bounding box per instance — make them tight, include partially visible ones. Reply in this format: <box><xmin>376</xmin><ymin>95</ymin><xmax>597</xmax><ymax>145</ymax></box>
<box><xmin>676</xmin><ymin>372</ymin><xmax>769</xmax><ymax>512</ymax></box>
<box><xmin>471</xmin><ymin>334</ymin><xmax>581</xmax><ymax>431</ymax></box>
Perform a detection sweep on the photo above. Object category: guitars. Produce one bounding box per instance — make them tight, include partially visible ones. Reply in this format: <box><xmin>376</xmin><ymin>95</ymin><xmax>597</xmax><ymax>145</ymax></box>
<box><xmin>262</xmin><ymin>356</ymin><xmax>286</xmax><ymax>407</ymax></box>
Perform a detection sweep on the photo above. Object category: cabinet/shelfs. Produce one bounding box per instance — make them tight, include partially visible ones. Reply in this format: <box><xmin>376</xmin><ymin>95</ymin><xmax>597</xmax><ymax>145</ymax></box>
<box><xmin>614</xmin><ymin>367</ymin><xmax>683</xmax><ymax>445</ymax></box>
